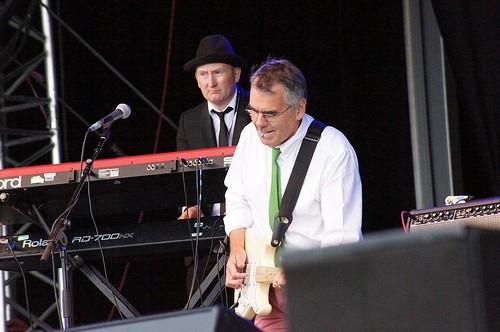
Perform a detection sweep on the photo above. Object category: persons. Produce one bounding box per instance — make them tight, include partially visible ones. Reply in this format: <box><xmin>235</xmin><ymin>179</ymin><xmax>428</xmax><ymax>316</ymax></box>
<box><xmin>216</xmin><ymin>55</ymin><xmax>362</xmax><ymax>332</ymax></box>
<box><xmin>173</xmin><ymin>35</ymin><xmax>254</xmax><ymax>306</ymax></box>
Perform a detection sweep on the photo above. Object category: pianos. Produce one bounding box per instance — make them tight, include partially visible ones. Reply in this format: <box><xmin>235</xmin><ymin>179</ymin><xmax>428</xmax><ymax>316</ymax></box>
<box><xmin>0</xmin><ymin>145</ymin><xmax>239</xmax><ymax>329</ymax></box>
<box><xmin>398</xmin><ymin>198</ymin><xmax>499</xmax><ymax>233</ymax></box>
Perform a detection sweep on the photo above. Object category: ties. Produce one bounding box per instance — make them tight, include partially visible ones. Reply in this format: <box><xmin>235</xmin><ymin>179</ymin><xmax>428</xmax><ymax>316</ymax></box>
<box><xmin>267</xmin><ymin>147</ymin><xmax>287</xmax><ymax>267</ymax></box>
<box><xmin>211</xmin><ymin>106</ymin><xmax>233</xmax><ymax>146</ymax></box>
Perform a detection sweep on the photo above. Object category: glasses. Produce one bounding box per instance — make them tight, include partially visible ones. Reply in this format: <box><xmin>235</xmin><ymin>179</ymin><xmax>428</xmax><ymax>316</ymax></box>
<box><xmin>245</xmin><ymin>102</ymin><xmax>291</xmax><ymax>119</ymax></box>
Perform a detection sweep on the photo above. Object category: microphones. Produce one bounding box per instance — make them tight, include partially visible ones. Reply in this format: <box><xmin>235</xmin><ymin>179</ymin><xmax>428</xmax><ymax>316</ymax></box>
<box><xmin>91</xmin><ymin>103</ymin><xmax>131</xmax><ymax>131</ymax></box>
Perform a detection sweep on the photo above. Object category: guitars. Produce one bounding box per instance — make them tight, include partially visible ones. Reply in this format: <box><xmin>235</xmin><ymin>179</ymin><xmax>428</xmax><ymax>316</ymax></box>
<box><xmin>231</xmin><ymin>229</ymin><xmax>288</xmax><ymax>320</ymax></box>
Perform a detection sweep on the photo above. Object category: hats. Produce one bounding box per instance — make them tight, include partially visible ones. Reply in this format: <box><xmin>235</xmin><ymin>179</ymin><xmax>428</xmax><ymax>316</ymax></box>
<box><xmin>182</xmin><ymin>34</ymin><xmax>250</xmax><ymax>76</ymax></box>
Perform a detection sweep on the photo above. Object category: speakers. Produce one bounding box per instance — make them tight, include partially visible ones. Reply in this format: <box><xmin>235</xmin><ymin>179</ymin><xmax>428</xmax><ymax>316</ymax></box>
<box><xmin>276</xmin><ymin>223</ymin><xmax>499</xmax><ymax>331</ymax></box>
